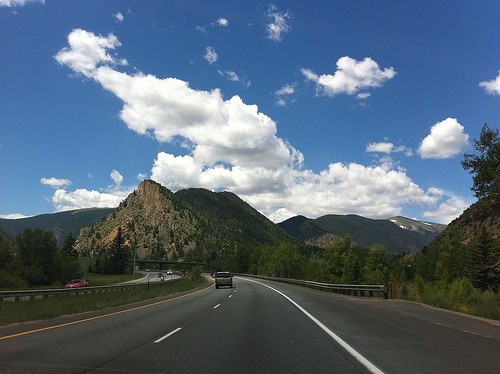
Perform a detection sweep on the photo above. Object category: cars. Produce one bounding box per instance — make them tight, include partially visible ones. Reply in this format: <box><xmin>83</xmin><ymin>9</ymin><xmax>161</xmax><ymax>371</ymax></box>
<box><xmin>145</xmin><ymin>269</ymin><xmax>150</xmax><ymax>272</ymax></box>
<box><xmin>64</xmin><ymin>279</ymin><xmax>88</xmax><ymax>287</ymax></box>
<box><xmin>157</xmin><ymin>273</ymin><xmax>163</xmax><ymax>277</ymax></box>
<box><xmin>211</xmin><ymin>273</ymin><xmax>216</xmax><ymax>278</ymax></box>
<box><xmin>167</xmin><ymin>270</ymin><xmax>173</xmax><ymax>275</ymax></box>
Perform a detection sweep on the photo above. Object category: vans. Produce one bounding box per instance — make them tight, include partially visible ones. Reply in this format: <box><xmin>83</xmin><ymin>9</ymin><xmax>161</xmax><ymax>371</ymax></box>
<box><xmin>215</xmin><ymin>271</ymin><xmax>233</xmax><ymax>288</ymax></box>
